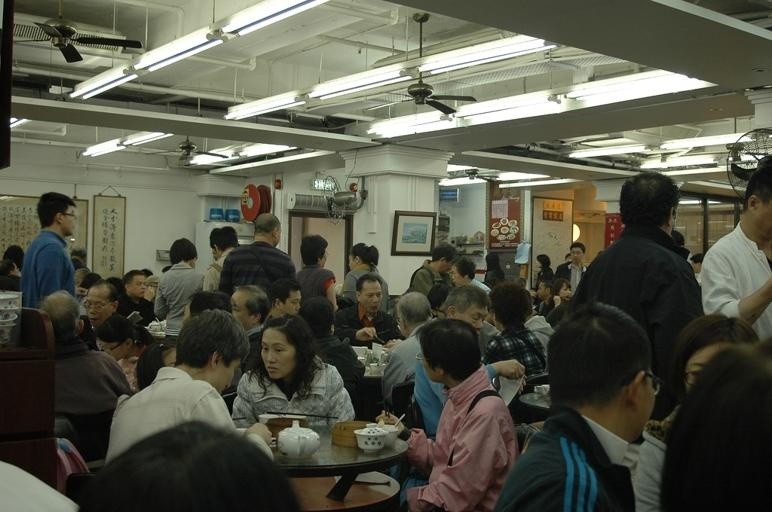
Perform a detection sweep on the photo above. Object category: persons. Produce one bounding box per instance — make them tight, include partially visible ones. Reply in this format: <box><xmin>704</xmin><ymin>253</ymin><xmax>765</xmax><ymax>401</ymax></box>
<box><xmin>0</xmin><ymin>154</ymin><xmax>772</xmax><ymax>511</ymax></box>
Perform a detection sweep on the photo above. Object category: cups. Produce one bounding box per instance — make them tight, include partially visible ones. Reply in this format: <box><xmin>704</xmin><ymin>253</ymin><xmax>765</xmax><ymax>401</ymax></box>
<box><xmin>353</xmin><ymin>428</ymin><xmax>387</xmax><ymax>453</ymax></box>
<box><xmin>148</xmin><ymin>323</ymin><xmax>161</xmax><ymax>332</ymax></box>
<box><xmin>235</xmin><ymin>427</ymin><xmax>248</xmax><ymax>437</ymax></box>
<box><xmin>1</xmin><ymin>291</ymin><xmax>20</xmax><ymax>346</ymax></box>
<box><xmin>353</xmin><ymin>346</ymin><xmax>389</xmax><ymax>375</ymax></box>
<box><xmin>541</xmin><ymin>385</ymin><xmax>550</xmax><ymax>395</ymax></box>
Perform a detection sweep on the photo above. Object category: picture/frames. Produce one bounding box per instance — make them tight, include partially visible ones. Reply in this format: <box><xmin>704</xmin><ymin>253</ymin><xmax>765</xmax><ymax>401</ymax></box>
<box><xmin>1</xmin><ymin>193</ymin><xmax>90</xmax><ymax>255</ymax></box>
<box><xmin>390</xmin><ymin>209</ymin><xmax>437</xmax><ymax>257</ymax></box>
<box><xmin>92</xmin><ymin>193</ymin><xmax>127</xmax><ymax>277</ymax></box>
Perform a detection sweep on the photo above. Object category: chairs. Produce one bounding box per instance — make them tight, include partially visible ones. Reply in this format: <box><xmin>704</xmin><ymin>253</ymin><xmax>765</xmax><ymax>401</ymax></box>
<box><xmin>390</xmin><ymin>381</ymin><xmax>415</xmax><ymax>420</ymax></box>
<box><xmin>520</xmin><ymin>372</ymin><xmax>549</xmax><ymax>393</ymax></box>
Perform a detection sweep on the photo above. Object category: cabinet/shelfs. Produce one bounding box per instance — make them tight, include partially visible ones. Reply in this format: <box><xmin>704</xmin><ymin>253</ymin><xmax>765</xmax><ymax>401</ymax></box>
<box><xmin>455</xmin><ymin>241</ymin><xmax>483</xmax><ymax>257</ymax></box>
<box><xmin>0</xmin><ymin>307</ymin><xmax>60</xmax><ymax>492</ymax></box>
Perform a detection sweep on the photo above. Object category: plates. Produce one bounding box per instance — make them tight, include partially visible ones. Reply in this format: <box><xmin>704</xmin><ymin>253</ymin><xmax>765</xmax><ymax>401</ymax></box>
<box><xmin>490</xmin><ymin>217</ymin><xmax>519</xmax><ymax>242</ymax></box>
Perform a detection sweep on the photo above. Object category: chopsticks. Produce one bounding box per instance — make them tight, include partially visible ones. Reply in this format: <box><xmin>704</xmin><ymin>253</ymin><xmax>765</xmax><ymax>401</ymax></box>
<box><xmin>267</xmin><ymin>411</ymin><xmax>339</xmax><ymax>419</ymax></box>
<box><xmin>384</xmin><ymin>407</ymin><xmax>390</xmax><ymax>417</ymax></box>
<box><xmin>230</xmin><ymin>416</ymin><xmax>249</xmax><ymax>421</ymax></box>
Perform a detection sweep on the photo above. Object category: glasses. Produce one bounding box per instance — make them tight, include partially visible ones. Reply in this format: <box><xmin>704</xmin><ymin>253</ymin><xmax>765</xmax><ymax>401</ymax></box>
<box><xmin>416</xmin><ymin>353</ymin><xmax>427</xmax><ymax>361</ymax></box>
<box><xmin>623</xmin><ymin>368</ymin><xmax>663</xmax><ymax>395</ymax></box>
<box><xmin>84</xmin><ymin>301</ymin><xmax>109</xmax><ymax>313</ymax></box>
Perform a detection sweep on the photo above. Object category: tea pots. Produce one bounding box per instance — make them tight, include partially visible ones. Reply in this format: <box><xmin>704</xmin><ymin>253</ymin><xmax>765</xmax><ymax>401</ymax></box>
<box><xmin>278</xmin><ymin>420</ymin><xmax>321</xmax><ymax>460</ymax></box>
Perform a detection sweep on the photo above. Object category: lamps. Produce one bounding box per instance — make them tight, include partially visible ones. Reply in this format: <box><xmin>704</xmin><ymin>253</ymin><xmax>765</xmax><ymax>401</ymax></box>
<box><xmin>562</xmin><ymin>69</ymin><xmax>690</xmax><ymax>101</ymax></box>
<box><xmin>223</xmin><ymin>91</ymin><xmax>304</xmax><ymax>120</ymax></box>
<box><xmin>223</xmin><ymin>149</ymin><xmax>335</xmax><ymax>173</ymax></box>
<box><xmin>80</xmin><ymin>131</ymin><xmax>173</xmax><ymax>160</ymax></box>
<box><xmin>188</xmin><ymin>141</ymin><xmax>300</xmax><ymax>169</ymax></box>
<box><xmin>66</xmin><ymin>0</ymin><xmax>331</xmax><ymax>101</ymax></box>
<box><xmin>658</xmin><ymin>133</ymin><xmax>752</xmax><ymax>150</ymax></box>
<box><xmin>640</xmin><ymin>154</ymin><xmax>719</xmax><ymax>168</ymax></box>
<box><xmin>568</xmin><ymin>143</ymin><xmax>647</xmax><ymax>159</ymax></box>
<box><xmin>436</xmin><ymin>170</ymin><xmax>552</xmax><ymax>186</ymax></box>
<box><xmin>307</xmin><ymin>66</ymin><xmax>417</xmax><ymax>103</ymax></box>
<box><xmin>366</xmin><ymin>108</ymin><xmax>458</xmax><ymax>136</ymax></box>
<box><xmin>499</xmin><ymin>178</ymin><xmax>584</xmax><ymax>187</ymax></box>
<box><xmin>457</xmin><ymin>90</ymin><xmax>549</xmax><ymax>115</ymax></box>
<box><xmin>415</xmin><ymin>34</ymin><xmax>559</xmax><ymax>75</ymax></box>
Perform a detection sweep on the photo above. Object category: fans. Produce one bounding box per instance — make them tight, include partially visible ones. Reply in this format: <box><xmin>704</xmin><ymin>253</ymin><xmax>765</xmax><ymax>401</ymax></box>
<box><xmin>726</xmin><ymin>127</ymin><xmax>772</xmax><ymax>200</ymax></box>
<box><xmin>13</xmin><ymin>0</ymin><xmax>143</xmax><ymax>64</ymax></box>
<box><xmin>322</xmin><ymin>175</ymin><xmax>369</xmax><ymax>224</ymax></box>
<box><xmin>365</xmin><ymin>11</ymin><xmax>478</xmax><ymax>115</ymax></box>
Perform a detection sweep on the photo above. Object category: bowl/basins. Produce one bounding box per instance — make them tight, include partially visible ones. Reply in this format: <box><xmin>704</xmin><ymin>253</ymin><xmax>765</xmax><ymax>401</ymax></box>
<box><xmin>377</xmin><ymin>423</ymin><xmax>399</xmax><ymax>447</ymax></box>
<box><xmin>258</xmin><ymin>413</ymin><xmax>309</xmax><ymax>437</ymax></box>
